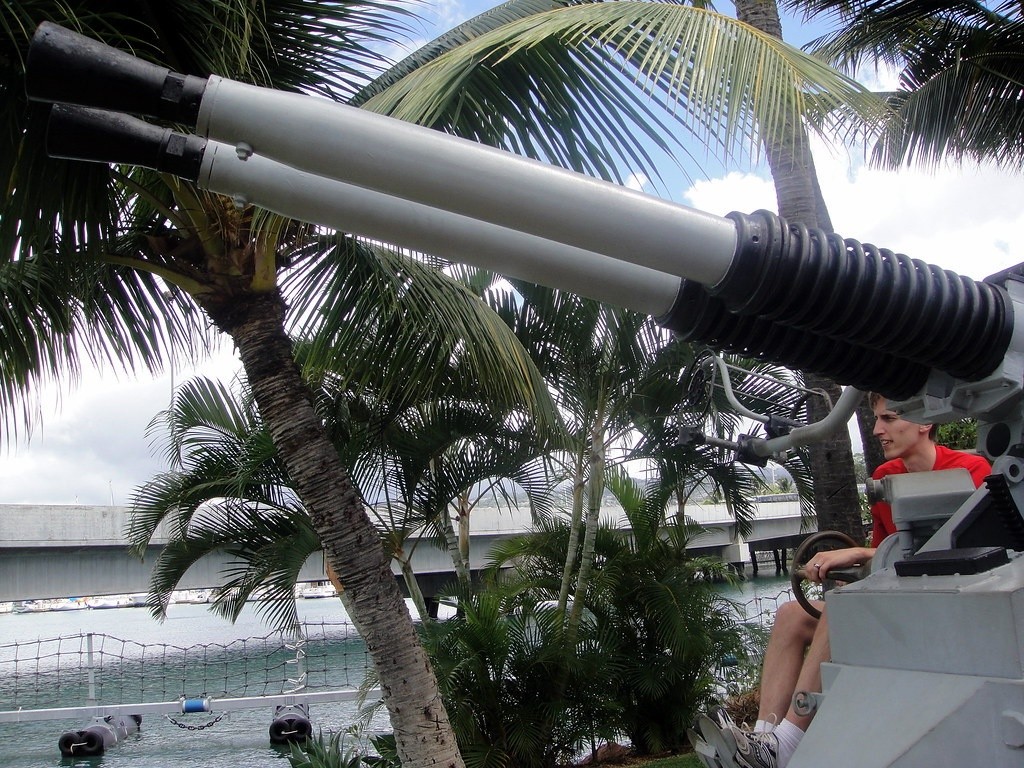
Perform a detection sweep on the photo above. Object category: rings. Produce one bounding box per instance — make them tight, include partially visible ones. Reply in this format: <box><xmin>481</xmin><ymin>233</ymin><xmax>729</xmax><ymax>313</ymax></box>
<box><xmin>814</xmin><ymin>563</ymin><xmax>820</xmax><ymax>570</ymax></box>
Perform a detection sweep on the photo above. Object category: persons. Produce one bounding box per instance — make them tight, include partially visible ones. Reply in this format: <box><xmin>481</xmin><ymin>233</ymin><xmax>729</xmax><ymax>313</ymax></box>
<box><xmin>706</xmin><ymin>387</ymin><xmax>994</xmax><ymax>768</ymax></box>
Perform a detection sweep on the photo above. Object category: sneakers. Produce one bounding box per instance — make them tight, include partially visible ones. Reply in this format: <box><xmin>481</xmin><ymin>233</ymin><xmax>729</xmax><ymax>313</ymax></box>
<box><xmin>707</xmin><ymin>705</ymin><xmax>779</xmax><ymax>768</ymax></box>
<box><xmin>693</xmin><ymin>714</ymin><xmax>719</xmax><ymax>756</ymax></box>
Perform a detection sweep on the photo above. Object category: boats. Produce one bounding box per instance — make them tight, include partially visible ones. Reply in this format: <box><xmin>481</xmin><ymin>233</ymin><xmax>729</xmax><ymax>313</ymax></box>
<box><xmin>0</xmin><ymin>581</ymin><xmax>339</xmax><ymax>613</ymax></box>
